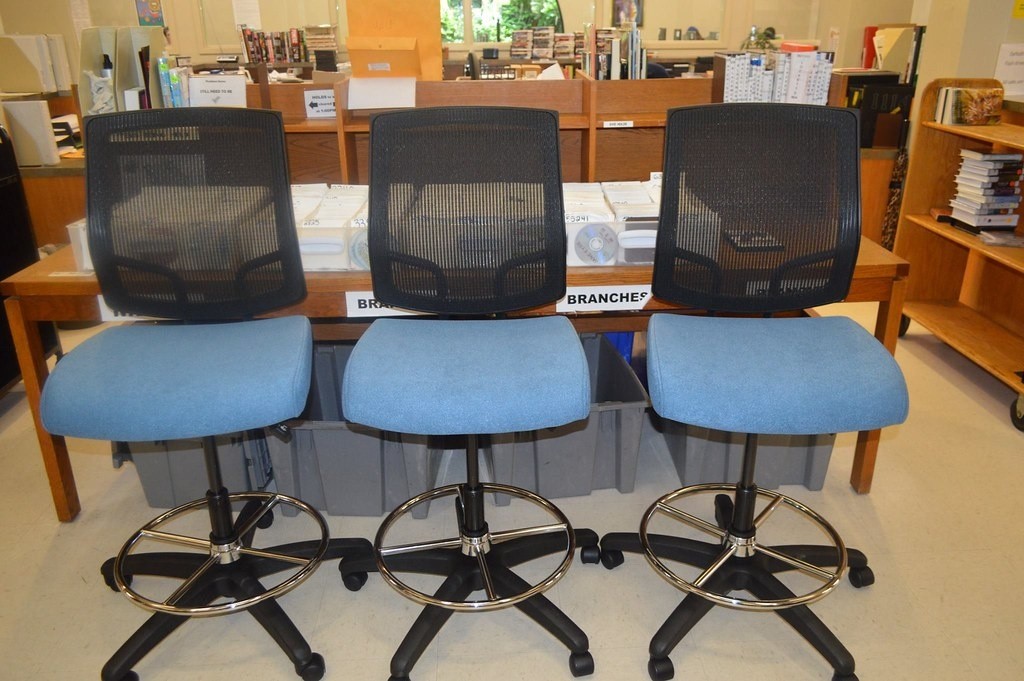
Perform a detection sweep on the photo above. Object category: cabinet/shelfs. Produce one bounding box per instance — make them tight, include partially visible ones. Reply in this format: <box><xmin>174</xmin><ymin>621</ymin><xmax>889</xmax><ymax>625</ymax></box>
<box><xmin>892</xmin><ymin>78</ymin><xmax>1024</xmax><ymax>432</ymax></box>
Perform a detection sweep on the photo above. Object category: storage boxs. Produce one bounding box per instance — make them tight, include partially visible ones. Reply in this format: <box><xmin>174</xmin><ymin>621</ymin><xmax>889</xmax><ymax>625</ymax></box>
<box><xmin>663</xmin><ymin>418</ymin><xmax>837</xmax><ymax>489</ymax></box>
<box><xmin>261</xmin><ymin>343</ymin><xmax>444</xmax><ymax>520</ymax></box>
<box><xmin>484</xmin><ymin>330</ymin><xmax>651</xmax><ymax>507</ymax></box>
<box><xmin>109</xmin><ymin>422</ymin><xmax>272</xmax><ymax>511</ymax></box>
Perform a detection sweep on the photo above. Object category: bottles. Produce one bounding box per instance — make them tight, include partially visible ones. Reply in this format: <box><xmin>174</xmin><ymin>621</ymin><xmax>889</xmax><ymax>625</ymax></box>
<box><xmin>751</xmin><ymin>25</ymin><xmax>758</xmax><ymax>40</ymax></box>
<box><xmin>100</xmin><ymin>54</ymin><xmax>113</xmax><ymax>78</ymax></box>
<box><xmin>464</xmin><ymin>64</ymin><xmax>470</xmax><ymax>76</ymax></box>
<box><xmin>477</xmin><ymin>32</ymin><xmax>488</xmax><ymax>42</ymax></box>
<box><xmin>443</xmin><ymin>45</ymin><xmax>449</xmax><ymax>59</ymax></box>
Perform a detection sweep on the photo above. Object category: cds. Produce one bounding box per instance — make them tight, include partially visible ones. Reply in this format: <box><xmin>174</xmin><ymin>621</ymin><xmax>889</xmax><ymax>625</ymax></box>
<box><xmin>575</xmin><ymin>223</ymin><xmax>618</xmax><ymax>265</ymax></box>
<box><xmin>349</xmin><ymin>230</ymin><xmax>371</xmax><ymax>270</ymax></box>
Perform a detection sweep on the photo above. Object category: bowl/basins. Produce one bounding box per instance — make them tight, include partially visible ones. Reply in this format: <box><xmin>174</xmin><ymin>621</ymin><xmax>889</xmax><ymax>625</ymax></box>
<box><xmin>484</xmin><ymin>49</ymin><xmax>499</xmax><ymax>58</ymax></box>
<box><xmin>709</xmin><ymin>32</ymin><xmax>719</xmax><ymax>40</ymax></box>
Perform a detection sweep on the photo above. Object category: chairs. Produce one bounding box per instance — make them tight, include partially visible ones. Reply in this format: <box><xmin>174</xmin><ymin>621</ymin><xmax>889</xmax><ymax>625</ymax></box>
<box><xmin>596</xmin><ymin>104</ymin><xmax>910</xmax><ymax>681</ymax></box>
<box><xmin>38</xmin><ymin>108</ymin><xmax>372</xmax><ymax>681</ymax></box>
<box><xmin>339</xmin><ymin>106</ymin><xmax>602</xmax><ymax>681</ymax></box>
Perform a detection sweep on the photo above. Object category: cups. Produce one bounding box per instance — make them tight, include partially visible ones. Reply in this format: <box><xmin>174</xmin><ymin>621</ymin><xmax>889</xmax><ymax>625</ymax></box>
<box><xmin>657</xmin><ymin>27</ymin><xmax>666</xmax><ymax>40</ymax></box>
<box><xmin>674</xmin><ymin>29</ymin><xmax>681</xmax><ymax>40</ymax></box>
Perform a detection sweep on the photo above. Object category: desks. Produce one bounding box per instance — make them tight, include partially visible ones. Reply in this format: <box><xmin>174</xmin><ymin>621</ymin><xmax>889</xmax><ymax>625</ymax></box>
<box><xmin>0</xmin><ymin>184</ymin><xmax>911</xmax><ymax>523</ymax></box>
